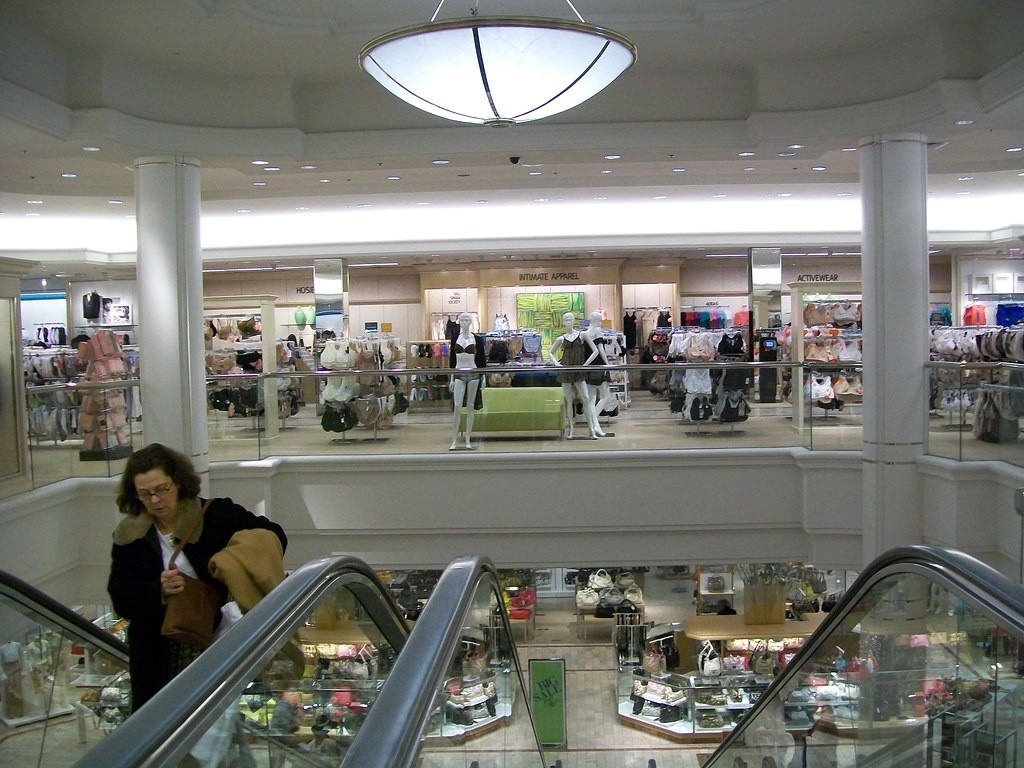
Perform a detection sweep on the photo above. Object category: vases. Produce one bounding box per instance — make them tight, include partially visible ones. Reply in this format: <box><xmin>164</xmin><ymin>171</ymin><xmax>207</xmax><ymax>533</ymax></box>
<box><xmin>294</xmin><ymin>305</ymin><xmax>316</xmax><ymax>324</ymax></box>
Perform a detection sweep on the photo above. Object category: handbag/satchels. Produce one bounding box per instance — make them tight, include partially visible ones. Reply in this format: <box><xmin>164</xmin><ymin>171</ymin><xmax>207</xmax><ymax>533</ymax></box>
<box><xmin>80</xmin><ymin>560</ymin><xmax>1010</xmax><ymax>743</ymax></box>
<box><xmin>161</xmin><ymin>498</ymin><xmax>224</xmax><ymax>646</ymax></box>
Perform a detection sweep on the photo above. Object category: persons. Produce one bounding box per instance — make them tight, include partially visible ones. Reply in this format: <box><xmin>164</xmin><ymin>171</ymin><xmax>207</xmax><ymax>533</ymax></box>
<box><xmin>102</xmin><ymin>298</ymin><xmax>114</xmax><ymax>323</ymax></box>
<box><xmin>586</xmin><ymin>312</ymin><xmax>614</xmax><ymax>436</ymax></box>
<box><xmin>745</xmin><ymin>695</ymin><xmax>794</xmax><ymax>768</ymax></box>
<box><xmin>0</xmin><ymin>641</ymin><xmax>26</xmax><ymax>719</ymax></box>
<box><xmin>268</xmin><ymin>692</ymin><xmax>297</xmax><ymax>768</ymax></box>
<box><xmin>27</xmin><ymin>636</ymin><xmax>54</xmax><ymax>694</ymax></box>
<box><xmin>309</xmin><ymin>715</ymin><xmax>339</xmax><ymax>755</ymax></box>
<box><xmin>105</xmin><ymin>443</ymin><xmax>289</xmax><ymax>717</ymax></box>
<box><xmin>549</xmin><ymin>312</ymin><xmax>599</xmax><ymax>440</ymax></box>
<box><xmin>809</xmin><ymin>705</ymin><xmax>839</xmax><ymax>768</ymax></box>
<box><xmin>450</xmin><ymin>312</ymin><xmax>486</xmax><ymax>449</ymax></box>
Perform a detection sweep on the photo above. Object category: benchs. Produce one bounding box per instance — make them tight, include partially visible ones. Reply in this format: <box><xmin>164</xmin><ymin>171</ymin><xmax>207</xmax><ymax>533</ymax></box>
<box><xmin>458</xmin><ymin>387</ymin><xmax>568</xmax><ymax>442</ymax></box>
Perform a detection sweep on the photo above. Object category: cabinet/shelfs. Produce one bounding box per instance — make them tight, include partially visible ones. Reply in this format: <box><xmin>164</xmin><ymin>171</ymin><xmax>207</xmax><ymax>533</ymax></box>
<box><xmin>488</xmin><ymin>568</ymin><xmax>538</xmax><ymax>639</ymax></box>
<box><xmin>788</xmin><ymin>281</ymin><xmax>1016</xmax><ymax>437</ymax></box>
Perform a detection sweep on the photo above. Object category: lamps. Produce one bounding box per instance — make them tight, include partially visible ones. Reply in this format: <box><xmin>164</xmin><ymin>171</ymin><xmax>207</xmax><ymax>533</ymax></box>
<box><xmin>357</xmin><ymin>0</ymin><xmax>638</xmax><ymax>129</ymax></box>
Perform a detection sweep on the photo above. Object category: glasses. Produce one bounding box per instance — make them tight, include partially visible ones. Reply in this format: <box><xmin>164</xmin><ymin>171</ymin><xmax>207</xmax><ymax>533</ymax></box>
<box><xmin>134</xmin><ymin>479</ymin><xmax>173</xmax><ymax>500</ymax></box>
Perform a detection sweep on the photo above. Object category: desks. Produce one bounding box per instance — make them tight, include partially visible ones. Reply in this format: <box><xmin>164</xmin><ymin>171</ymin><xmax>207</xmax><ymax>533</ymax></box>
<box><xmin>574</xmin><ymin>569</ymin><xmax>647</xmax><ymax>639</ymax></box>
